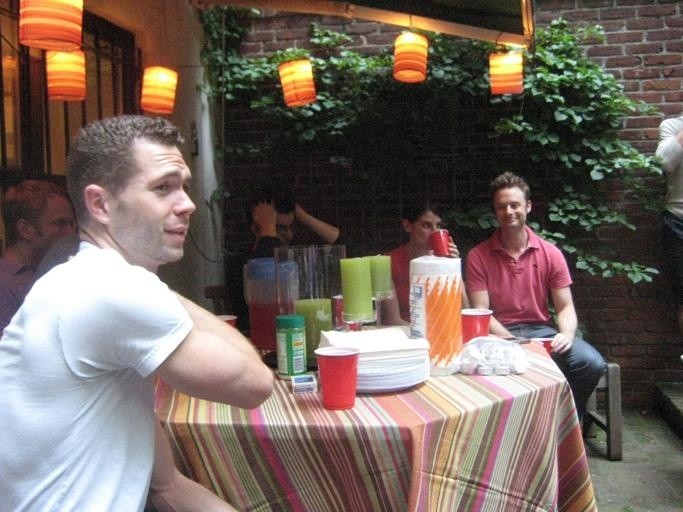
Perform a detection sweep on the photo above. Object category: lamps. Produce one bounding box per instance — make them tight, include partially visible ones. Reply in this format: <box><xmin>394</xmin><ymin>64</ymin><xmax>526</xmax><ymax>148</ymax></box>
<box><xmin>138</xmin><ymin>64</ymin><xmax>179</xmax><ymax>115</ymax></box>
<box><xmin>45</xmin><ymin>46</ymin><xmax>89</xmax><ymax>103</ymax></box>
<box><xmin>15</xmin><ymin>0</ymin><xmax>84</xmax><ymax>52</ymax></box>
<box><xmin>486</xmin><ymin>27</ymin><xmax>523</xmax><ymax>98</ymax></box>
<box><xmin>275</xmin><ymin>8</ymin><xmax>319</xmax><ymax>111</ymax></box>
<box><xmin>392</xmin><ymin>12</ymin><xmax>429</xmax><ymax>83</ymax></box>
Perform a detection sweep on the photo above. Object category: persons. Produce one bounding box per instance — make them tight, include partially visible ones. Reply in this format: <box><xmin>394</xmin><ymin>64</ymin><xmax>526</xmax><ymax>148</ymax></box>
<box><xmin>0</xmin><ymin>115</ymin><xmax>275</xmax><ymax>512</ymax></box>
<box><xmin>463</xmin><ymin>170</ymin><xmax>606</xmax><ymax>440</ymax></box>
<box><xmin>656</xmin><ymin>114</ymin><xmax>683</xmax><ymax>363</ymax></box>
<box><xmin>0</xmin><ymin>179</ymin><xmax>74</xmax><ymax>342</ymax></box>
<box><xmin>378</xmin><ymin>204</ymin><xmax>470</xmax><ymax>328</ymax></box>
<box><xmin>232</xmin><ymin>186</ymin><xmax>355</xmax><ymax>336</ymax></box>
<box><xmin>32</xmin><ymin>228</ymin><xmax>79</xmax><ymax>281</ymax></box>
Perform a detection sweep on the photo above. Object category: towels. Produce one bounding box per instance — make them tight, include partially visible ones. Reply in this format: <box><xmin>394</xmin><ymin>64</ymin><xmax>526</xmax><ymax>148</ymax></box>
<box><xmin>314</xmin><ymin>328</ymin><xmax>430</xmax><ymax>378</ymax></box>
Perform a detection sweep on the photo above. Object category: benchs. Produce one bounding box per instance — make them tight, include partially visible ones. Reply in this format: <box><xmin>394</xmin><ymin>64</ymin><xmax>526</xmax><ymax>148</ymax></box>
<box><xmin>203</xmin><ymin>281</ymin><xmax>623</xmax><ymax>462</ymax></box>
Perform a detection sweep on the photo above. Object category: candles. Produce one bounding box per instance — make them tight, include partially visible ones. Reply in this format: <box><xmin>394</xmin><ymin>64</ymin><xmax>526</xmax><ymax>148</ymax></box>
<box><xmin>362</xmin><ymin>253</ymin><xmax>393</xmax><ymax>300</ymax></box>
<box><xmin>337</xmin><ymin>255</ymin><xmax>375</xmax><ymax>325</ymax></box>
<box><xmin>293</xmin><ymin>292</ymin><xmax>333</xmax><ymax>370</ymax></box>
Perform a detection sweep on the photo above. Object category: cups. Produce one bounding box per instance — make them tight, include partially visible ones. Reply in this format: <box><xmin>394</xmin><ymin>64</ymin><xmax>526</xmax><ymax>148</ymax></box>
<box><xmin>461</xmin><ymin>307</ymin><xmax>494</xmax><ymax>343</ymax></box>
<box><xmin>215</xmin><ymin>245</ymin><xmax>360</xmax><ymax>409</ymax></box>
<box><xmin>532</xmin><ymin>337</ymin><xmax>553</xmax><ymax>360</ymax></box>
<box><xmin>429</xmin><ymin>228</ymin><xmax>451</xmax><ymax>258</ymax></box>
